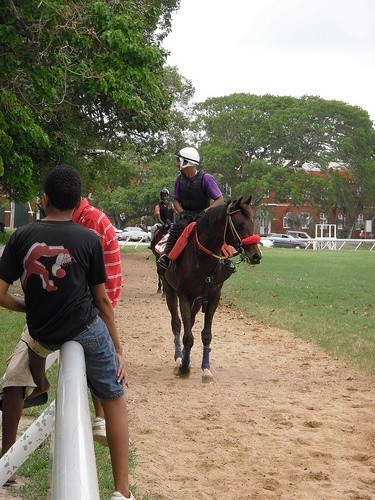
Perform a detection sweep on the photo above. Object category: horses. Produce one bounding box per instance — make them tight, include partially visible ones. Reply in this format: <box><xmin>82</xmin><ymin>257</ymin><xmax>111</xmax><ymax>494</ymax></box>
<box><xmin>161</xmin><ymin>195</ymin><xmax>262</xmax><ymax>382</ymax></box>
<box><xmin>151</xmin><ymin>224</ymin><xmax>173</xmax><ymax>297</ymax></box>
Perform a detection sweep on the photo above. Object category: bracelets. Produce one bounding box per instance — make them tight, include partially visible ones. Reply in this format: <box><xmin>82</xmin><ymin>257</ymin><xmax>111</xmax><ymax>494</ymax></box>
<box><xmin>203</xmin><ymin>210</ymin><xmax>207</xmax><ymax>214</ymax></box>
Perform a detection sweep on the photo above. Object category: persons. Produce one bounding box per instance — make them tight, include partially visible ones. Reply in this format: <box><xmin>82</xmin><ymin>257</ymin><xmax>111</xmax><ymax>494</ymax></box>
<box><xmin>148</xmin><ymin>187</ymin><xmax>179</xmax><ymax>249</ymax></box>
<box><xmin>158</xmin><ymin>147</ymin><xmax>236</xmax><ymax>268</ymax></box>
<box><xmin>360</xmin><ymin>229</ymin><xmax>365</xmax><ymax>243</ymax></box>
<box><xmin>0</xmin><ymin>196</ymin><xmax>121</xmax><ymax>483</ymax></box>
<box><xmin>0</xmin><ymin>168</ymin><xmax>136</xmax><ymax>500</ymax></box>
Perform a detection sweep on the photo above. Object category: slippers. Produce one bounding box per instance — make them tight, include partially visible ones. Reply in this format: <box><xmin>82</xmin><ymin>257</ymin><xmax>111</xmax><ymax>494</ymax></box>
<box><xmin>0</xmin><ymin>390</ymin><xmax>48</xmax><ymax>411</ymax></box>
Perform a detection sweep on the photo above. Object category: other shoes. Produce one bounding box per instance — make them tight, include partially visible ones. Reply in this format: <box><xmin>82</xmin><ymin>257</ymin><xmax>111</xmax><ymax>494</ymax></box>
<box><xmin>157</xmin><ymin>258</ymin><xmax>167</xmax><ymax>275</ymax></box>
<box><xmin>92</xmin><ymin>416</ymin><xmax>107</xmax><ymax>445</ymax></box>
<box><xmin>110</xmin><ymin>490</ymin><xmax>135</xmax><ymax>500</ymax></box>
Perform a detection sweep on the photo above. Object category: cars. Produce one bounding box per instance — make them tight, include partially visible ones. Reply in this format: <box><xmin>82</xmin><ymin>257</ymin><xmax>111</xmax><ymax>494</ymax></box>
<box><xmin>116</xmin><ymin>227</ymin><xmax>149</xmax><ymax>242</ymax></box>
<box><xmin>256</xmin><ymin>230</ymin><xmax>313</xmax><ymax>250</ymax></box>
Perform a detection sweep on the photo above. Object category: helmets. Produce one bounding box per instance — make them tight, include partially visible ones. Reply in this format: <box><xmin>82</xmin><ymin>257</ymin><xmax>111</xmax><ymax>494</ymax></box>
<box><xmin>173</xmin><ymin>146</ymin><xmax>200</xmax><ymax>167</ymax></box>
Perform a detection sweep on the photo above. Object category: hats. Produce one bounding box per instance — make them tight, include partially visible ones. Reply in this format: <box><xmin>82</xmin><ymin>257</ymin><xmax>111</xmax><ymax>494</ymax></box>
<box><xmin>160</xmin><ymin>189</ymin><xmax>170</xmax><ymax>195</ymax></box>
